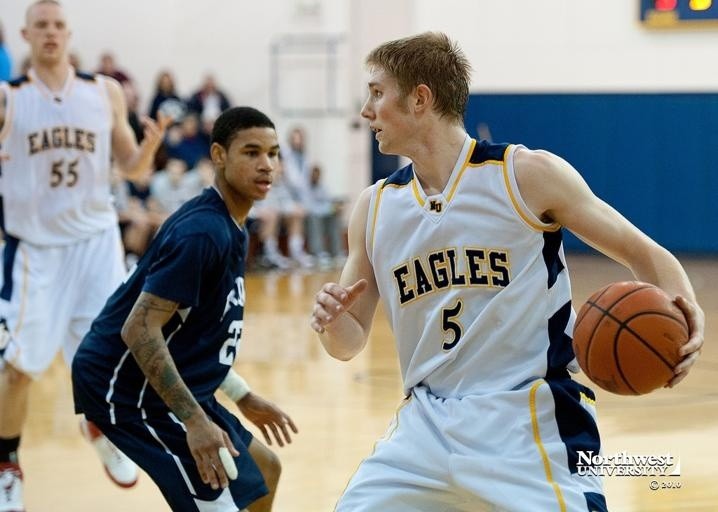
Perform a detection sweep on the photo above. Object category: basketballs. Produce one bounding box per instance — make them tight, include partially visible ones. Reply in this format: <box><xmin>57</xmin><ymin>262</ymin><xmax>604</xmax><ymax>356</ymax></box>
<box><xmin>572</xmin><ymin>281</ymin><xmax>688</xmax><ymax>397</ymax></box>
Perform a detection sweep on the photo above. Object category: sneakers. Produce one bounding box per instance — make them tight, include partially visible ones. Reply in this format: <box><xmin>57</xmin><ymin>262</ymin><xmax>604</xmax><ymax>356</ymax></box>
<box><xmin>79</xmin><ymin>419</ymin><xmax>142</xmax><ymax>489</ymax></box>
<box><xmin>0</xmin><ymin>462</ymin><xmax>23</xmax><ymax>512</ymax></box>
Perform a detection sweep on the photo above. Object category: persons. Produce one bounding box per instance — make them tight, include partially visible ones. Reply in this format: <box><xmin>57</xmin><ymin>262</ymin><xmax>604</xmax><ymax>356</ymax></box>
<box><xmin>72</xmin><ymin>105</ymin><xmax>300</xmax><ymax>511</ymax></box>
<box><xmin>308</xmin><ymin>28</ymin><xmax>707</xmax><ymax>511</ymax></box>
<box><xmin>2</xmin><ymin>47</ymin><xmax>350</xmax><ymax>349</ymax></box>
<box><xmin>0</xmin><ymin>0</ymin><xmax>182</xmax><ymax>510</ymax></box>
